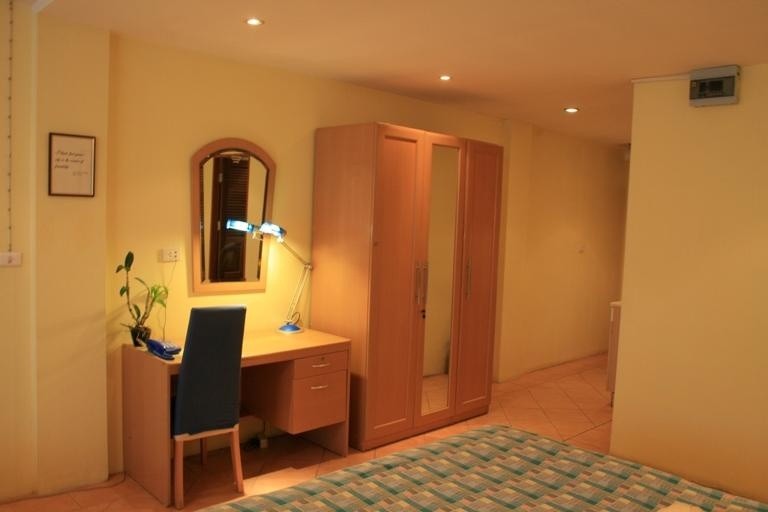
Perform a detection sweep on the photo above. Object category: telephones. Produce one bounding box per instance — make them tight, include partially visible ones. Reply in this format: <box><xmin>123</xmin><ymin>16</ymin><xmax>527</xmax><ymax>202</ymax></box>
<box><xmin>146</xmin><ymin>339</ymin><xmax>181</xmax><ymax>354</ymax></box>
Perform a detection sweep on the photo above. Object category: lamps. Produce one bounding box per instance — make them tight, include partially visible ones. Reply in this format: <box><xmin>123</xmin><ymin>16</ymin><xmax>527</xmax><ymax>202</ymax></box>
<box><xmin>227</xmin><ymin>220</ymin><xmax>262</xmax><ymax>243</ymax></box>
<box><xmin>261</xmin><ymin>221</ymin><xmax>312</xmax><ymax>333</ymax></box>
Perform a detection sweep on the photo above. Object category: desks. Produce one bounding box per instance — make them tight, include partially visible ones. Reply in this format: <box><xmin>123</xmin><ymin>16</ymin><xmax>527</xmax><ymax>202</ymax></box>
<box><xmin>122</xmin><ymin>326</ymin><xmax>352</xmax><ymax>507</ymax></box>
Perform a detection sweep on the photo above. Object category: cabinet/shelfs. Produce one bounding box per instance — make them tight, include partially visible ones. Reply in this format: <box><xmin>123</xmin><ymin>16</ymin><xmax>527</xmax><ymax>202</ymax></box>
<box><xmin>309</xmin><ymin>123</ymin><xmax>504</xmax><ymax>452</ymax></box>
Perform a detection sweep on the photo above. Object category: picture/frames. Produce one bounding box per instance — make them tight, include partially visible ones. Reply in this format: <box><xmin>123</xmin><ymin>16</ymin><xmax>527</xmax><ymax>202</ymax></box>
<box><xmin>49</xmin><ymin>132</ymin><xmax>96</xmax><ymax>197</ymax></box>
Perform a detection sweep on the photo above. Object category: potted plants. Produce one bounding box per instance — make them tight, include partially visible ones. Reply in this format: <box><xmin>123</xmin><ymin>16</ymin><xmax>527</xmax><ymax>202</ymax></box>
<box><xmin>115</xmin><ymin>251</ymin><xmax>168</xmax><ymax>347</ymax></box>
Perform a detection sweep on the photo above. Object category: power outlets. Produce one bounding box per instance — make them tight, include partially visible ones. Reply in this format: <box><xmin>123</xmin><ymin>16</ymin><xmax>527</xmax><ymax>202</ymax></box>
<box><xmin>161</xmin><ymin>249</ymin><xmax>179</xmax><ymax>262</ymax></box>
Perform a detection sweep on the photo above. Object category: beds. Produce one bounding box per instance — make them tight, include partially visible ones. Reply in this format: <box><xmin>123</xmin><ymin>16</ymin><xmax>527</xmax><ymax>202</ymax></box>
<box><xmin>203</xmin><ymin>420</ymin><xmax>767</xmax><ymax>510</ymax></box>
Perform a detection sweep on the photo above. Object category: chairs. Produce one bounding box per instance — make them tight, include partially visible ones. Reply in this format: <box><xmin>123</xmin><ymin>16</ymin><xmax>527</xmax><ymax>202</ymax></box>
<box><xmin>171</xmin><ymin>305</ymin><xmax>247</xmax><ymax>509</ymax></box>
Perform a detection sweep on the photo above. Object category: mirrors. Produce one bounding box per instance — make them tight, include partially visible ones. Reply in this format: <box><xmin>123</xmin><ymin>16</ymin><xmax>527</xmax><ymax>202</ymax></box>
<box><xmin>421</xmin><ymin>144</ymin><xmax>461</xmax><ymax>416</ymax></box>
<box><xmin>192</xmin><ymin>138</ymin><xmax>277</xmax><ymax>295</ymax></box>
